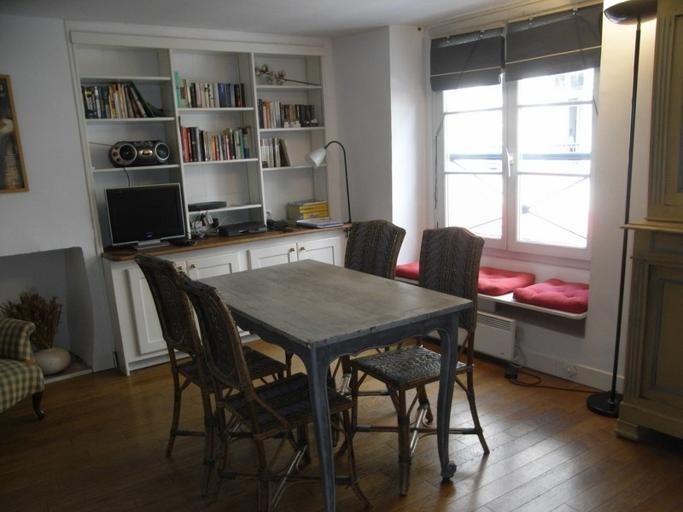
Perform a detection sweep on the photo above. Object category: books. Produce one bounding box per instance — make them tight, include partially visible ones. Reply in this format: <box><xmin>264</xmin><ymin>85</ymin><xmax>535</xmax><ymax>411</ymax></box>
<box><xmin>176</xmin><ymin>74</ymin><xmax>325</xmax><ymax>169</ymax></box>
<box><xmin>82</xmin><ymin>79</ymin><xmax>171</xmax><ymax>117</ymax></box>
<box><xmin>285</xmin><ymin>198</ymin><xmax>345</xmax><ymax>230</ymax></box>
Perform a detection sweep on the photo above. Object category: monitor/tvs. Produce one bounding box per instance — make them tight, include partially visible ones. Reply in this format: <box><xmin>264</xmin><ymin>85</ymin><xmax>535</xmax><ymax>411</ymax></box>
<box><xmin>104</xmin><ymin>183</ymin><xmax>185</xmax><ymax>250</ymax></box>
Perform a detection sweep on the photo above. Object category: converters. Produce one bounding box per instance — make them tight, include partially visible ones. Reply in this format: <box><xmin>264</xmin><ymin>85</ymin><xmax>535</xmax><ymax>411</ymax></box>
<box><xmin>504</xmin><ymin>368</ymin><xmax>518</xmax><ymax>378</ymax></box>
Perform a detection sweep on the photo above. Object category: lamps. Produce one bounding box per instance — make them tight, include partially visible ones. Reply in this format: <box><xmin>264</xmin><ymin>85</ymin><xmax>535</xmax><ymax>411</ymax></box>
<box><xmin>587</xmin><ymin>0</ymin><xmax>657</xmax><ymax>420</ymax></box>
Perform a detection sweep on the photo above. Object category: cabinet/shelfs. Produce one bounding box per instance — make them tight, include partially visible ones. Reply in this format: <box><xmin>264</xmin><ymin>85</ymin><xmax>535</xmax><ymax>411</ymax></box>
<box><xmin>614</xmin><ymin>0</ymin><xmax>683</xmax><ymax>441</ymax></box>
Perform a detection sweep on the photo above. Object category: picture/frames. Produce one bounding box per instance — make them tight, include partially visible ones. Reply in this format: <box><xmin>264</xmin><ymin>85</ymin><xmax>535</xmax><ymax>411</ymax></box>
<box><xmin>0</xmin><ymin>73</ymin><xmax>30</xmax><ymax>193</ymax></box>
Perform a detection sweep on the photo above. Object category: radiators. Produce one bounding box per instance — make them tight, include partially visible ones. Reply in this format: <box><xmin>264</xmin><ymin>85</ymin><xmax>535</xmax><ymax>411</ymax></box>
<box><xmin>427</xmin><ymin>309</ymin><xmax>518</xmax><ymax>363</ymax></box>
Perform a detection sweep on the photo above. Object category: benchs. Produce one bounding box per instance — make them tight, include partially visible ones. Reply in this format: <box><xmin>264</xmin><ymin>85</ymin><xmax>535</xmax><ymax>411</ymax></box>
<box><xmin>395</xmin><ymin>275</ymin><xmax>587</xmax><ymax>387</ymax></box>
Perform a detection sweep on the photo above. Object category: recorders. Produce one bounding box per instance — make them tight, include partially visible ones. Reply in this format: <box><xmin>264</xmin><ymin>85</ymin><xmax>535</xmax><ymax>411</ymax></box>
<box><xmin>109</xmin><ymin>141</ymin><xmax>171</xmax><ymax>168</ymax></box>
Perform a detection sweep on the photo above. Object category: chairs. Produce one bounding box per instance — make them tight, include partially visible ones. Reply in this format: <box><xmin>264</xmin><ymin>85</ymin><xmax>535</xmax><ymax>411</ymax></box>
<box><xmin>0</xmin><ymin>316</ymin><xmax>48</xmax><ymax>420</ymax></box>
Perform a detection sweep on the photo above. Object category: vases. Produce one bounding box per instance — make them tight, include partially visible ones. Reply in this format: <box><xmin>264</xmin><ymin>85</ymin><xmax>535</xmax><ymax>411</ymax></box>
<box><xmin>34</xmin><ymin>347</ymin><xmax>71</xmax><ymax>376</ymax></box>
